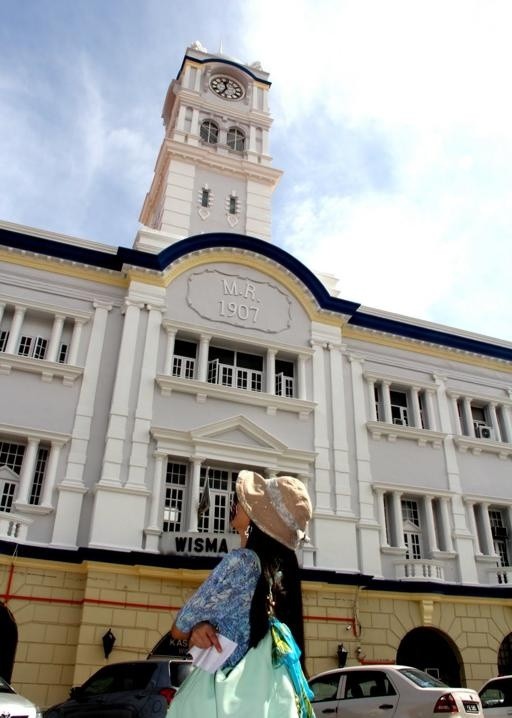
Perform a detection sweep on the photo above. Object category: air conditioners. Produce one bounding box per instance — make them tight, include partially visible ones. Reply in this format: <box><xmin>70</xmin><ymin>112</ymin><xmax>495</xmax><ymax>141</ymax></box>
<box><xmin>392</xmin><ymin>418</ymin><xmax>406</xmax><ymax>427</ymax></box>
<box><xmin>474</xmin><ymin>425</ymin><xmax>492</xmax><ymax>441</ymax></box>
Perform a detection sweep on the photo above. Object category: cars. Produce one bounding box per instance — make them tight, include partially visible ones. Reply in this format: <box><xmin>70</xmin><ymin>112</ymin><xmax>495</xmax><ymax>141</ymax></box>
<box><xmin>477</xmin><ymin>674</ymin><xmax>512</xmax><ymax>718</ymax></box>
<box><xmin>0</xmin><ymin>674</ymin><xmax>41</xmax><ymax>718</ymax></box>
<box><xmin>303</xmin><ymin>662</ymin><xmax>485</xmax><ymax>718</ymax></box>
<box><xmin>41</xmin><ymin>658</ymin><xmax>200</xmax><ymax>718</ymax></box>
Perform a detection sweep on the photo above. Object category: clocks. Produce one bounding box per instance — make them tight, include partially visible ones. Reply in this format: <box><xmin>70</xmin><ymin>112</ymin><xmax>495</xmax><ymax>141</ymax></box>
<box><xmin>208</xmin><ymin>73</ymin><xmax>245</xmax><ymax>101</ymax></box>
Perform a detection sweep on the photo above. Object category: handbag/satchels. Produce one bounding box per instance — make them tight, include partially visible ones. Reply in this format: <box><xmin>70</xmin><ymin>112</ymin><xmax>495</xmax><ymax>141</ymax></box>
<box><xmin>165</xmin><ymin>569</ymin><xmax>317</xmax><ymax>718</ymax></box>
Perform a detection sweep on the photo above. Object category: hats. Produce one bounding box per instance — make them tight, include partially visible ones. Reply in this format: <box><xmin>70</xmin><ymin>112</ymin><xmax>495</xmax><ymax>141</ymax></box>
<box><xmin>235</xmin><ymin>469</ymin><xmax>313</xmax><ymax>552</ymax></box>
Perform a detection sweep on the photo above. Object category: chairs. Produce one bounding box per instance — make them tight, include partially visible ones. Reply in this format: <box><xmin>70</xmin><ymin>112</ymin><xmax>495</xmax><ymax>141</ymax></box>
<box><xmin>369</xmin><ymin>686</ymin><xmax>385</xmax><ymax>695</ymax></box>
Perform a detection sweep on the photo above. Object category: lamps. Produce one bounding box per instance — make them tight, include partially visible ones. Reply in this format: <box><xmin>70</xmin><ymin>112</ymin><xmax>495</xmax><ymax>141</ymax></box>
<box><xmin>102</xmin><ymin>627</ymin><xmax>115</xmax><ymax>660</ymax></box>
<box><xmin>336</xmin><ymin>643</ymin><xmax>348</xmax><ymax>669</ymax></box>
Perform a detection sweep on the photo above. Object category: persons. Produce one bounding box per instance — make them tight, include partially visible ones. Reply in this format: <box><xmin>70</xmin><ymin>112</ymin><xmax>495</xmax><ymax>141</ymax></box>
<box><xmin>167</xmin><ymin>469</ymin><xmax>316</xmax><ymax>718</ymax></box>
